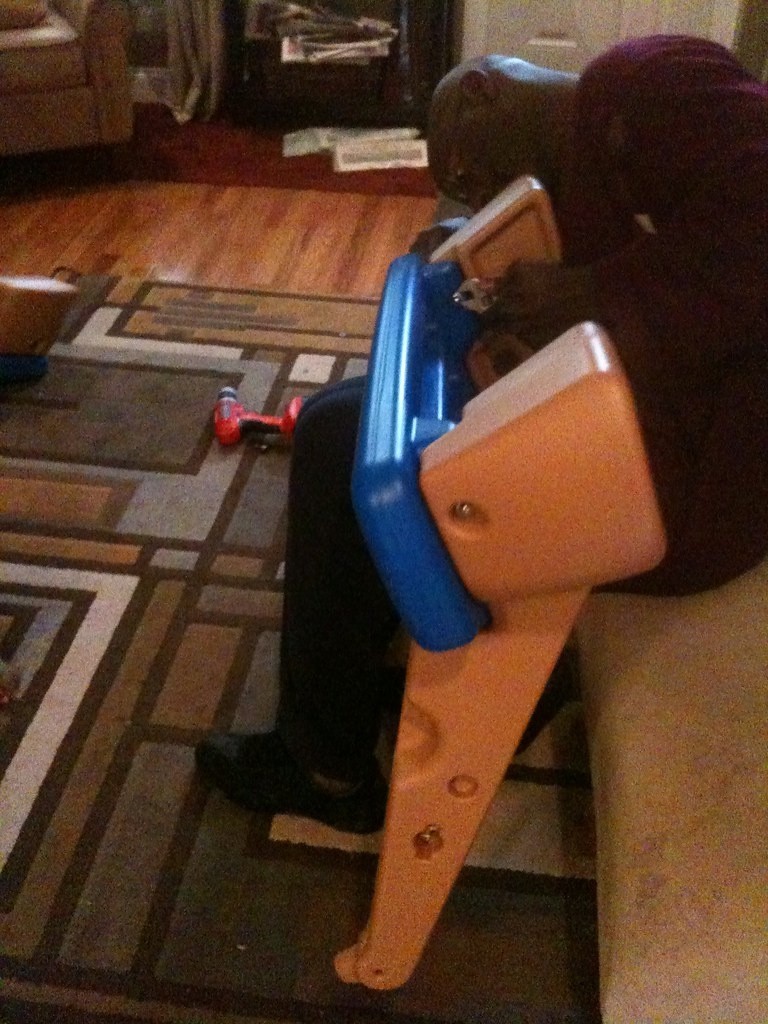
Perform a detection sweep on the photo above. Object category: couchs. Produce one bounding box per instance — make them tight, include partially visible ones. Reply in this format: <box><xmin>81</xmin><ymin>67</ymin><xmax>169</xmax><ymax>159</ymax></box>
<box><xmin>0</xmin><ymin>0</ymin><xmax>135</xmax><ymax>160</ymax></box>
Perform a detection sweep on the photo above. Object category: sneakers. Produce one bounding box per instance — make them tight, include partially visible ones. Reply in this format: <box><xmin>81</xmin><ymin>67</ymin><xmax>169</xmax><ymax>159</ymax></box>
<box><xmin>194</xmin><ymin>730</ymin><xmax>388</xmax><ymax>835</ymax></box>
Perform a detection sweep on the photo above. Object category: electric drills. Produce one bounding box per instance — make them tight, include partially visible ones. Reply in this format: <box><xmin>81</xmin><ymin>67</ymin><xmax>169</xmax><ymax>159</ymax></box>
<box><xmin>213</xmin><ymin>386</ymin><xmax>309</xmax><ymax>447</ymax></box>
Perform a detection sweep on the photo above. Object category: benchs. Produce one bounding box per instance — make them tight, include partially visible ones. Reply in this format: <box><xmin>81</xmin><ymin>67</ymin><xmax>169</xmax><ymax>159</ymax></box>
<box><xmin>581</xmin><ymin>563</ymin><xmax>768</xmax><ymax>1024</ymax></box>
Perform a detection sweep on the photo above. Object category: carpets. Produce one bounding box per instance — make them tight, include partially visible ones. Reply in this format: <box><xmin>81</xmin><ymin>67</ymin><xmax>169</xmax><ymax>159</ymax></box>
<box><xmin>40</xmin><ymin>102</ymin><xmax>436</xmax><ymax>198</ymax></box>
<box><xmin>0</xmin><ymin>273</ymin><xmax>600</xmax><ymax>1024</ymax></box>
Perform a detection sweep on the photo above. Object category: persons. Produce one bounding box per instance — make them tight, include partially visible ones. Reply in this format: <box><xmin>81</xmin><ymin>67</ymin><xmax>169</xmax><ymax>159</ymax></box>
<box><xmin>194</xmin><ymin>35</ymin><xmax>768</xmax><ymax>834</ymax></box>
